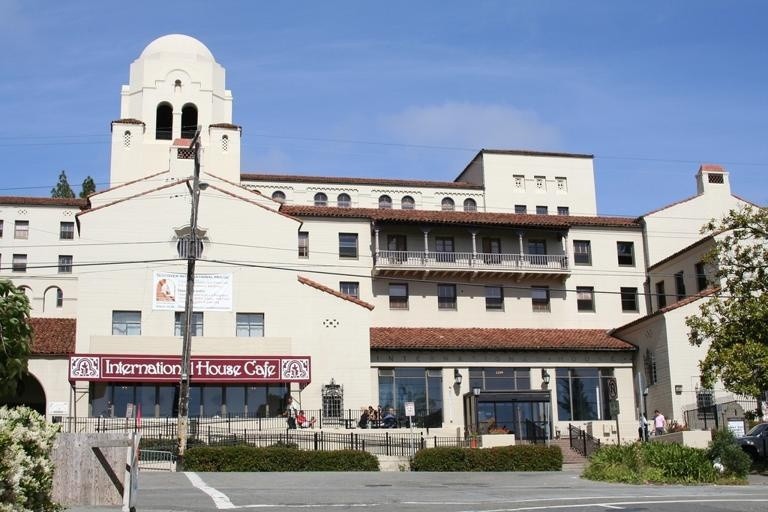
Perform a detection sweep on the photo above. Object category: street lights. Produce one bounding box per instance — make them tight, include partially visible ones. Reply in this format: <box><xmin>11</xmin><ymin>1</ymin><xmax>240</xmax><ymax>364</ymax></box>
<box><xmin>473</xmin><ymin>387</ymin><xmax>480</xmax><ymax>432</ymax></box>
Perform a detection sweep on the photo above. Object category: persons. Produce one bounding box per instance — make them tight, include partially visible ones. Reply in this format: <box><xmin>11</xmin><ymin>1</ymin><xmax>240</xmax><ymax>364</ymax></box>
<box><xmin>295</xmin><ymin>409</ymin><xmax>316</xmax><ymax>428</ymax></box>
<box><xmin>106</xmin><ymin>400</ymin><xmax>116</xmax><ymax>420</ymax></box>
<box><xmin>281</xmin><ymin>396</ymin><xmax>298</xmax><ymax>431</ymax></box>
<box><xmin>635</xmin><ymin>413</ymin><xmax>650</xmax><ymax>444</ymax></box>
<box><xmin>651</xmin><ymin>409</ymin><xmax>667</xmax><ymax>436</ymax></box>
<box><xmin>357</xmin><ymin>405</ymin><xmax>399</xmax><ymax>431</ymax></box>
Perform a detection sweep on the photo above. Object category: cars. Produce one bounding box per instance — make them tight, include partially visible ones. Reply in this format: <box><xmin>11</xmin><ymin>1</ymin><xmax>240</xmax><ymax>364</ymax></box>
<box><xmin>735</xmin><ymin>423</ymin><xmax>768</xmax><ymax>466</ymax></box>
